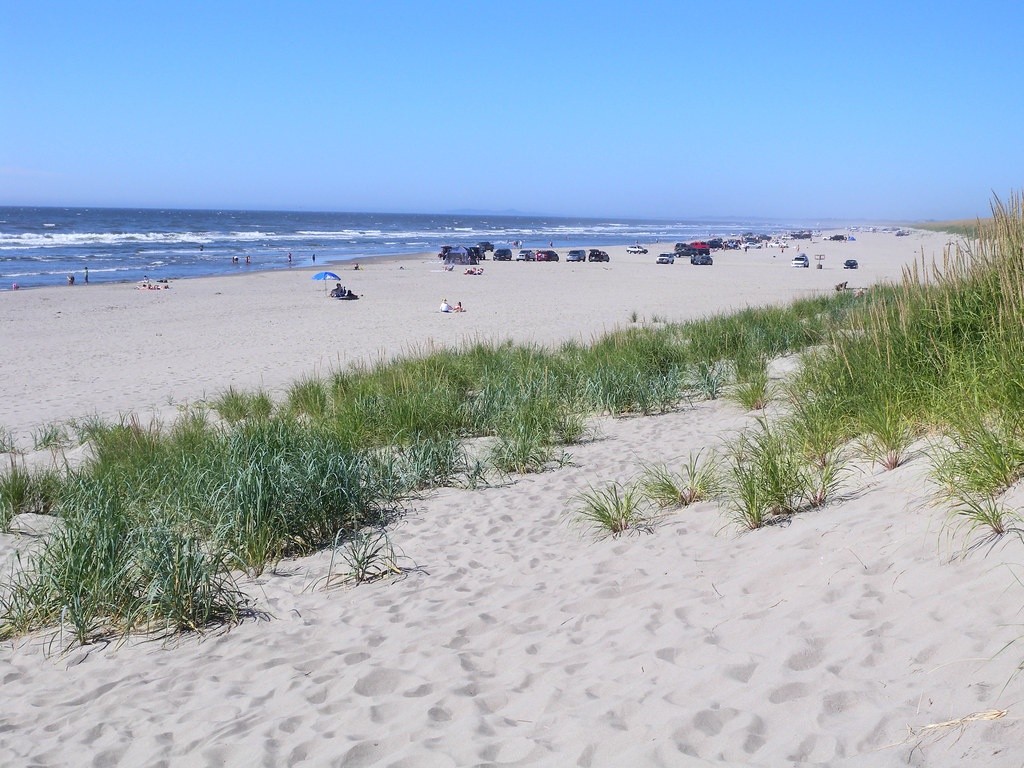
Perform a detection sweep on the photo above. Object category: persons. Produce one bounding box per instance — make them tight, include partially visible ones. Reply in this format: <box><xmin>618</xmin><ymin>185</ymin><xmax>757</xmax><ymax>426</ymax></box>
<box><xmin>464</xmin><ymin>268</ymin><xmax>484</xmax><ymax>275</ymax></box>
<box><xmin>69</xmin><ymin>275</ymin><xmax>74</xmax><ymax>285</ymax></box>
<box><xmin>329</xmin><ymin>283</ymin><xmax>354</xmax><ymax>299</ymax></box>
<box><xmin>354</xmin><ymin>263</ymin><xmax>359</xmax><ymax>270</ymax></box>
<box><xmin>440</xmin><ymin>299</ymin><xmax>463</xmax><ymax>312</ymax></box>
<box><xmin>445</xmin><ymin>264</ymin><xmax>454</xmax><ymax>271</ymax></box>
<box><xmin>13</xmin><ymin>283</ymin><xmax>17</xmax><ymax>290</ymax></box>
<box><xmin>312</xmin><ymin>254</ymin><xmax>315</xmax><ymax>261</ymax></box>
<box><xmin>550</xmin><ymin>240</ymin><xmax>552</xmax><ymax>247</ymax></box>
<box><xmin>85</xmin><ymin>267</ymin><xmax>88</xmax><ymax>285</ymax></box>
<box><xmin>138</xmin><ymin>275</ymin><xmax>169</xmax><ymax>290</ymax></box>
<box><xmin>200</xmin><ymin>243</ymin><xmax>203</xmax><ymax>251</ymax></box>
<box><xmin>289</xmin><ymin>252</ymin><xmax>291</xmax><ymax>262</ymax></box>
<box><xmin>506</xmin><ymin>239</ymin><xmax>522</xmax><ymax>249</ymax></box>
<box><xmin>233</xmin><ymin>256</ymin><xmax>238</xmax><ymax>264</ymax></box>
<box><xmin>245</xmin><ymin>255</ymin><xmax>250</xmax><ymax>262</ymax></box>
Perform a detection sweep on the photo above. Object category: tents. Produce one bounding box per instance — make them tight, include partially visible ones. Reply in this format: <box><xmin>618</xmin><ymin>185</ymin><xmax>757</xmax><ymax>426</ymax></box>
<box><xmin>444</xmin><ymin>244</ymin><xmax>477</xmax><ymax>265</ymax></box>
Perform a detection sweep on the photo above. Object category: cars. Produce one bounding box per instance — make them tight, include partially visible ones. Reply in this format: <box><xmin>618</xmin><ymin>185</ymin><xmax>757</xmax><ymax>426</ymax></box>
<box><xmin>437</xmin><ymin>229</ymin><xmax>846</xmax><ymax>265</ymax></box>
<box><xmin>843</xmin><ymin>260</ymin><xmax>858</xmax><ymax>269</ymax></box>
<box><xmin>791</xmin><ymin>256</ymin><xmax>809</xmax><ymax>268</ymax></box>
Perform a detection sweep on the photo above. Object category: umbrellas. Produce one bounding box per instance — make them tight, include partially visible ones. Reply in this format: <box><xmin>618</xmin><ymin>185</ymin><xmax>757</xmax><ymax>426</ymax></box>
<box><xmin>312</xmin><ymin>271</ymin><xmax>341</xmax><ymax>290</ymax></box>
